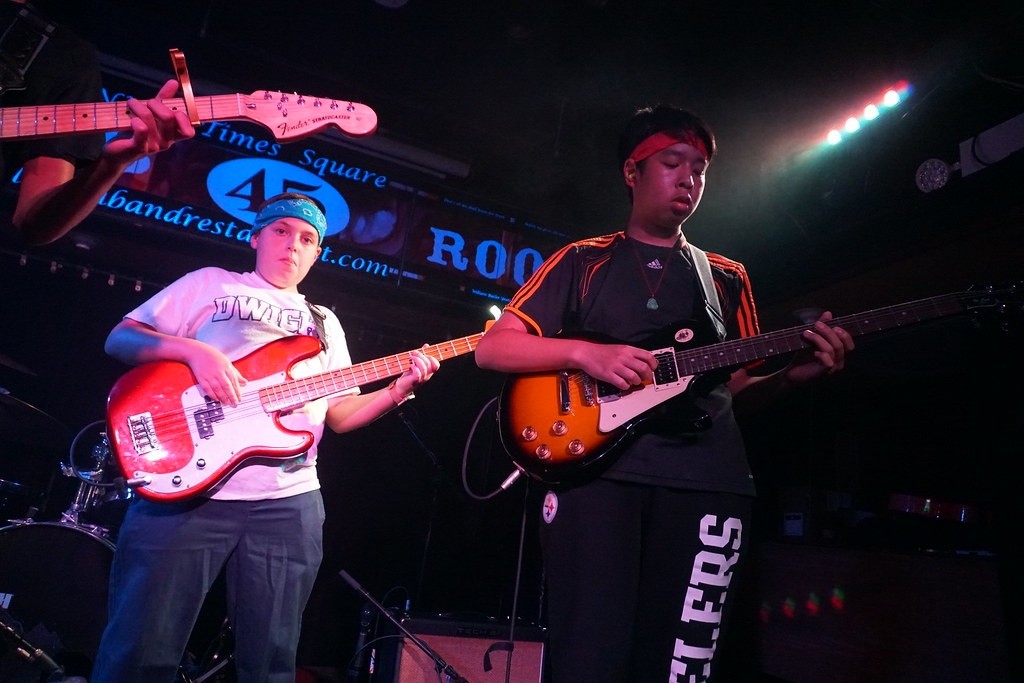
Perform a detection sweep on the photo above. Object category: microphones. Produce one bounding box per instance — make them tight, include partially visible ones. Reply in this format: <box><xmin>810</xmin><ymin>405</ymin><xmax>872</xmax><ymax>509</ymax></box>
<box><xmin>446</xmin><ymin>674</ymin><xmax>468</xmax><ymax>683</ymax></box>
<box><xmin>50</xmin><ymin>676</ymin><xmax>88</xmax><ymax>683</ymax></box>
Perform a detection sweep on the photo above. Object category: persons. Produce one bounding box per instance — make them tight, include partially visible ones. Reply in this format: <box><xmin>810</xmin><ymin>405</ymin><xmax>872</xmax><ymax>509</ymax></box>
<box><xmin>0</xmin><ymin>1</ymin><xmax>197</xmax><ymax>247</ymax></box>
<box><xmin>92</xmin><ymin>194</ymin><xmax>441</xmax><ymax>683</ymax></box>
<box><xmin>475</xmin><ymin>102</ymin><xmax>856</xmax><ymax>683</ymax></box>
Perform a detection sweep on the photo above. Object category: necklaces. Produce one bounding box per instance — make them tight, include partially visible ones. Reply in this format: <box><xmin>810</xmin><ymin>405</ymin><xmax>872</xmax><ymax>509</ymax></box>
<box><xmin>634</xmin><ymin>242</ymin><xmax>673</xmax><ymax>309</ymax></box>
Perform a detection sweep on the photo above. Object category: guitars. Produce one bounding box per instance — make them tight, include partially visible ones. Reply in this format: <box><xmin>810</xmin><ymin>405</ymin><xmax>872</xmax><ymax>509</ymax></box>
<box><xmin>99</xmin><ymin>317</ymin><xmax>498</xmax><ymax>519</ymax></box>
<box><xmin>495</xmin><ymin>278</ymin><xmax>1020</xmax><ymax>492</ymax></box>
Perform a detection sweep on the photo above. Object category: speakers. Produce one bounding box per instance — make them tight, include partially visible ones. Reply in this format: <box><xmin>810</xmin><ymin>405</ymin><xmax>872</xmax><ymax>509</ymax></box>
<box><xmin>378</xmin><ymin>611</ymin><xmax>545</xmax><ymax>683</ymax></box>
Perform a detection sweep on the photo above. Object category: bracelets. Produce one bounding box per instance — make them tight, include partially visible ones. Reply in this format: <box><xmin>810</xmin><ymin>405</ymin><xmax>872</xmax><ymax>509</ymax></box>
<box><xmin>388</xmin><ymin>379</ymin><xmax>406</xmax><ymax>406</ymax></box>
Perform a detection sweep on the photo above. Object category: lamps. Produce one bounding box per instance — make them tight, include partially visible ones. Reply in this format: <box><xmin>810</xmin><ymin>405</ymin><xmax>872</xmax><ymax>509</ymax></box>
<box><xmin>916</xmin><ymin>112</ymin><xmax>1024</xmax><ymax>192</ymax></box>
